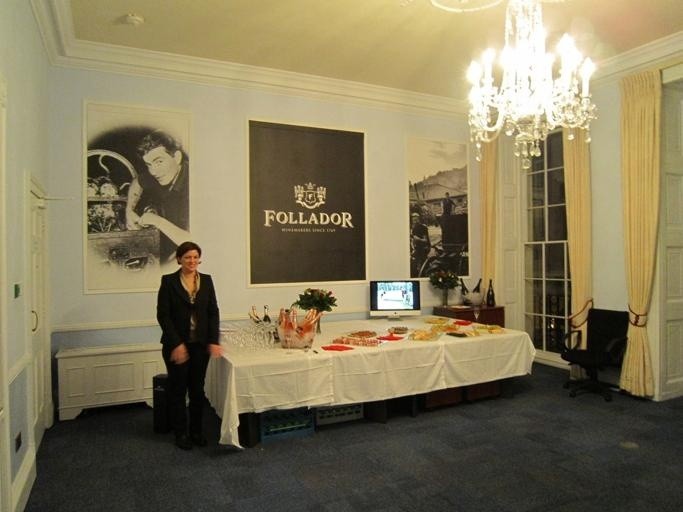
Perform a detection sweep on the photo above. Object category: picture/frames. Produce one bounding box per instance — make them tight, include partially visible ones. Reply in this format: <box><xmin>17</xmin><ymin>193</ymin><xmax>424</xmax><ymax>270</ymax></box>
<box><xmin>245</xmin><ymin>117</ymin><xmax>370</xmax><ymax>288</ymax></box>
<box><xmin>405</xmin><ymin>135</ymin><xmax>472</xmax><ymax>280</ymax></box>
<box><xmin>83</xmin><ymin>99</ymin><xmax>193</xmax><ymax>295</ymax></box>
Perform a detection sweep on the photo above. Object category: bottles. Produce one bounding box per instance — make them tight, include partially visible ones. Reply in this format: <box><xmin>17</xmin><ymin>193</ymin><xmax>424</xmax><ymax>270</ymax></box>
<box><xmin>248</xmin><ymin>304</ymin><xmax>271</xmax><ymax>322</ymax></box>
<box><xmin>460</xmin><ymin>278</ymin><xmax>468</xmax><ymax>294</ymax></box>
<box><xmin>473</xmin><ymin>278</ymin><xmax>482</xmax><ymax>293</ymax></box>
<box><xmin>487</xmin><ymin>279</ymin><xmax>494</xmax><ymax>307</ymax></box>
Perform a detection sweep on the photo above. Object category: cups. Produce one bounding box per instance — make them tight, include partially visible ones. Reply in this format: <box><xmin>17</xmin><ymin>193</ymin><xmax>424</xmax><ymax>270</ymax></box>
<box><xmin>232</xmin><ymin>322</ymin><xmax>274</xmax><ymax>354</ymax></box>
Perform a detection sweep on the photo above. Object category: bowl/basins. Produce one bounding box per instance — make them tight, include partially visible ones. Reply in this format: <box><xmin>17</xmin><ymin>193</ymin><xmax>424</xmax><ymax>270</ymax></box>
<box><xmin>463</xmin><ymin>293</ymin><xmax>484</xmax><ymax>306</ymax></box>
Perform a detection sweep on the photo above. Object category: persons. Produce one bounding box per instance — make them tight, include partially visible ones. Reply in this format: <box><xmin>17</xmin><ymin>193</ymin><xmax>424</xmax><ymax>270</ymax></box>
<box><xmin>410</xmin><ymin>192</ymin><xmax>457</xmax><ymax>265</ymax></box>
<box><xmin>126</xmin><ymin>130</ymin><xmax>191</xmax><ymax>246</ymax></box>
<box><xmin>157</xmin><ymin>241</ymin><xmax>223</xmax><ymax>450</ymax></box>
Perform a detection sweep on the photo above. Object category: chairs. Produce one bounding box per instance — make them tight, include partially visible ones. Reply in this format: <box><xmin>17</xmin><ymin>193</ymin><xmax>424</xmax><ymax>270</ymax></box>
<box><xmin>561</xmin><ymin>308</ymin><xmax>630</xmax><ymax>402</ymax></box>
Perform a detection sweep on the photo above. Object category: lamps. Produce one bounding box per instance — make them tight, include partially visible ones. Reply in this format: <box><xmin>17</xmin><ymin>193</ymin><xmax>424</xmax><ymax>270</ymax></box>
<box><xmin>464</xmin><ymin>0</ymin><xmax>601</xmax><ymax>170</ymax></box>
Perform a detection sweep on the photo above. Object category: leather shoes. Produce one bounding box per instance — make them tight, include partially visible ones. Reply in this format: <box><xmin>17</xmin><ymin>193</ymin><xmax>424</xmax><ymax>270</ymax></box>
<box><xmin>193</xmin><ymin>436</ymin><xmax>207</xmax><ymax>446</ymax></box>
<box><xmin>176</xmin><ymin>438</ymin><xmax>192</xmax><ymax>449</ymax></box>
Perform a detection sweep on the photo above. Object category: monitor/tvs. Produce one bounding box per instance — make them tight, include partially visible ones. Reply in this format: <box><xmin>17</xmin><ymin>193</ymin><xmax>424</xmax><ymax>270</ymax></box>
<box><xmin>370</xmin><ymin>280</ymin><xmax>421</xmax><ymax>319</ymax></box>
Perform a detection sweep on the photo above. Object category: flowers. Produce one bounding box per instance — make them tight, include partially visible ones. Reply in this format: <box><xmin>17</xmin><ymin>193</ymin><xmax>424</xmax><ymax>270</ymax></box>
<box><xmin>288</xmin><ymin>288</ymin><xmax>338</xmax><ymax>313</ymax></box>
<box><xmin>428</xmin><ymin>270</ymin><xmax>460</xmax><ymax>289</ymax></box>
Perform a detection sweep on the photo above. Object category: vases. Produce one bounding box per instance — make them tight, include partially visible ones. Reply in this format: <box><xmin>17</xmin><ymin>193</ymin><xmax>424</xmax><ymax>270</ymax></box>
<box><xmin>442</xmin><ymin>288</ymin><xmax>448</xmax><ymax>306</ymax></box>
<box><xmin>316</xmin><ymin>318</ymin><xmax>321</xmax><ymax>334</ymax></box>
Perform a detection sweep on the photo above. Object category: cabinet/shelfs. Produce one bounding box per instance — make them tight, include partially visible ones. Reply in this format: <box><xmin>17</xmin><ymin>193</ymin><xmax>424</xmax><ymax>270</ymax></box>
<box><xmin>433</xmin><ymin>304</ymin><xmax>506</xmax><ymax>330</ymax></box>
<box><xmin>55</xmin><ymin>343</ymin><xmax>168</xmax><ymax>421</ymax></box>
